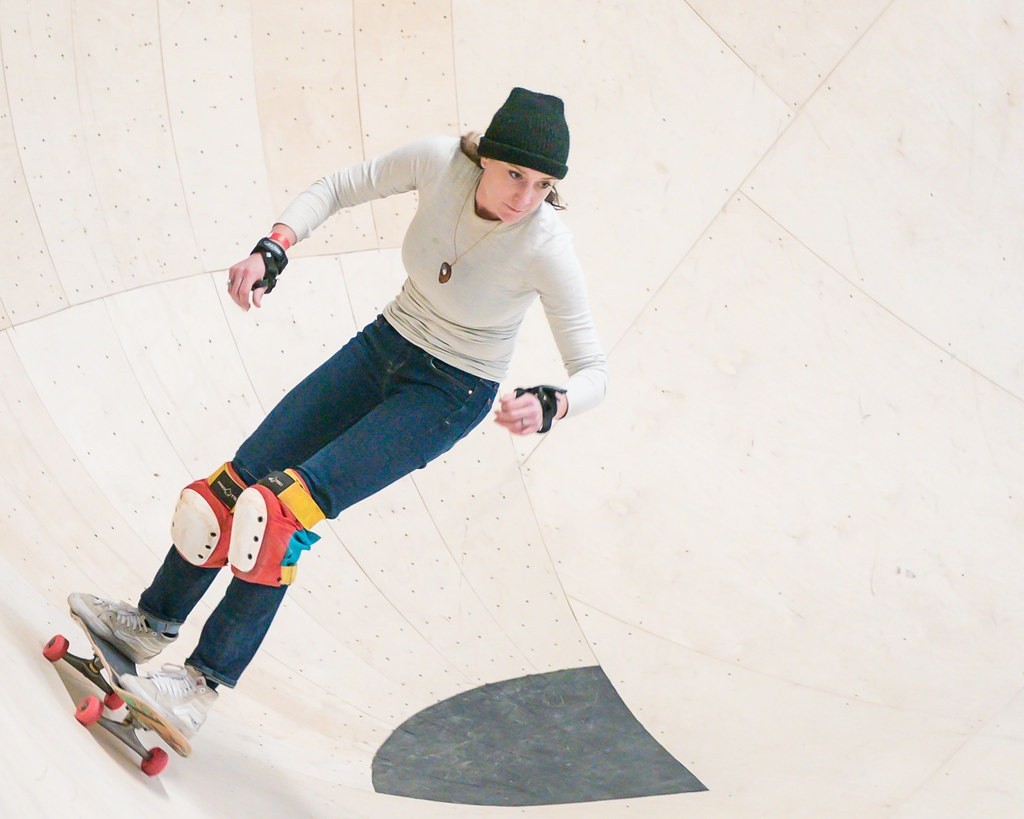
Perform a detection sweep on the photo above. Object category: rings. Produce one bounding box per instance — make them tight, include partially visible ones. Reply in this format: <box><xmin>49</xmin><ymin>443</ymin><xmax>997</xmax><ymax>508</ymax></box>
<box><xmin>522</xmin><ymin>419</ymin><xmax>526</xmax><ymax>428</ymax></box>
<box><xmin>227</xmin><ymin>278</ymin><xmax>234</xmax><ymax>285</ymax></box>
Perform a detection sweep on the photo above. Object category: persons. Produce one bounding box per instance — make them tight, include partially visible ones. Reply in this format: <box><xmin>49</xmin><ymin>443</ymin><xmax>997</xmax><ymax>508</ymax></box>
<box><xmin>67</xmin><ymin>87</ymin><xmax>607</xmax><ymax>739</ymax></box>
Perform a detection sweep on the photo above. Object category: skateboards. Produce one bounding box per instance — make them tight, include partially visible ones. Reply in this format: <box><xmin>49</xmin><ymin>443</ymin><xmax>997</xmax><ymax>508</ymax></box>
<box><xmin>42</xmin><ymin>609</ymin><xmax>170</xmax><ymax>777</ymax></box>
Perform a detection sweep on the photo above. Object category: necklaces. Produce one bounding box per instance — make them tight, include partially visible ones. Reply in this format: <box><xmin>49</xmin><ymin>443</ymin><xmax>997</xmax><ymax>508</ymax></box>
<box><xmin>438</xmin><ymin>171</ymin><xmax>504</xmax><ymax>283</ymax></box>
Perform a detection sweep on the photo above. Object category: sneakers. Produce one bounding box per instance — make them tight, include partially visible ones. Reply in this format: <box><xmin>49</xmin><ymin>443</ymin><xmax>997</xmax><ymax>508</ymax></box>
<box><xmin>118</xmin><ymin>663</ymin><xmax>220</xmax><ymax>742</ymax></box>
<box><xmin>68</xmin><ymin>593</ymin><xmax>179</xmax><ymax>664</ymax></box>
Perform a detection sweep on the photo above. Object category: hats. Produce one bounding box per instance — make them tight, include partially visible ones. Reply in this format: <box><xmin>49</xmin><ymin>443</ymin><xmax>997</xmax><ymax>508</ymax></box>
<box><xmin>477</xmin><ymin>87</ymin><xmax>570</xmax><ymax>179</ymax></box>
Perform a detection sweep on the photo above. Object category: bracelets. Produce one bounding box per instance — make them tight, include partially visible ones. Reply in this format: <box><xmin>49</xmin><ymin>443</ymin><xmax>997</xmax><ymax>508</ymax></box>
<box><xmin>249</xmin><ymin>237</ymin><xmax>288</xmax><ymax>275</ymax></box>
<box><xmin>515</xmin><ymin>385</ymin><xmax>567</xmax><ymax>434</ymax></box>
<box><xmin>267</xmin><ymin>232</ymin><xmax>290</xmax><ymax>250</ymax></box>
